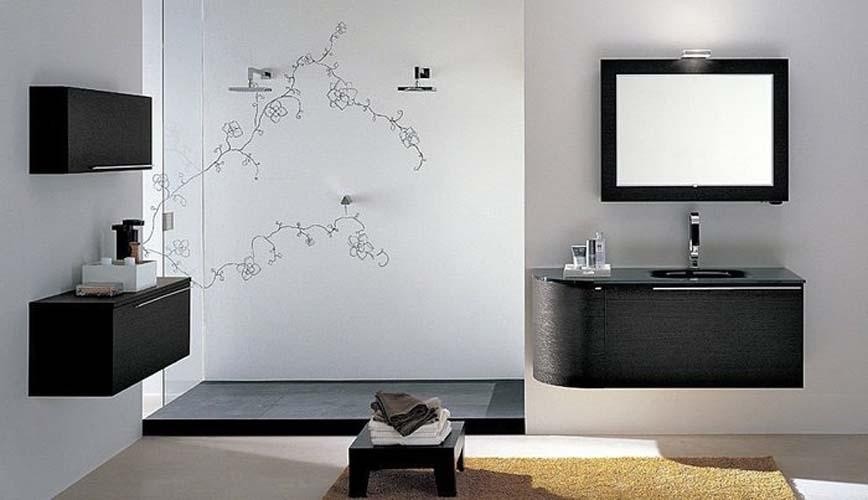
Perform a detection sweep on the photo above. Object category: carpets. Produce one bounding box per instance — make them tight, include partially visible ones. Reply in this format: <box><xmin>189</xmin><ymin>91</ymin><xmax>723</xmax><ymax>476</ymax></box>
<box><xmin>320</xmin><ymin>455</ymin><xmax>800</xmax><ymax>500</ymax></box>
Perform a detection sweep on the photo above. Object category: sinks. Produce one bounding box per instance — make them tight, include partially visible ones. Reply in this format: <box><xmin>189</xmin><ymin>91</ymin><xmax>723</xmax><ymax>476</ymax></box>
<box><xmin>649</xmin><ymin>267</ymin><xmax>748</xmax><ymax>280</ymax></box>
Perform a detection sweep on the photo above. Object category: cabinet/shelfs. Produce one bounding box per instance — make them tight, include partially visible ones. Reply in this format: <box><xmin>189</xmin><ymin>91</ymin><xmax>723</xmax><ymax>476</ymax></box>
<box><xmin>28</xmin><ymin>276</ymin><xmax>192</xmax><ymax>397</ymax></box>
<box><xmin>28</xmin><ymin>86</ymin><xmax>153</xmax><ymax>175</ymax></box>
<box><xmin>532</xmin><ymin>274</ymin><xmax>804</xmax><ymax>389</ymax></box>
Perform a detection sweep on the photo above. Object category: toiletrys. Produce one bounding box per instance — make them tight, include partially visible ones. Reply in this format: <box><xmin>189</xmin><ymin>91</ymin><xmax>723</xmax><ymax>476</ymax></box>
<box><xmin>571</xmin><ymin>245</ymin><xmax>585</xmax><ymax>266</ymax></box>
<box><xmin>572</xmin><ymin>232</ymin><xmax>607</xmax><ymax>269</ymax></box>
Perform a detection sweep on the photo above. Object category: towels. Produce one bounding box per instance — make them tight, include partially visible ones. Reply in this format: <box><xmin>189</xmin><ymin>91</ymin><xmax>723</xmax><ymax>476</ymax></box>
<box><xmin>368</xmin><ymin>391</ymin><xmax>452</xmax><ymax>446</ymax></box>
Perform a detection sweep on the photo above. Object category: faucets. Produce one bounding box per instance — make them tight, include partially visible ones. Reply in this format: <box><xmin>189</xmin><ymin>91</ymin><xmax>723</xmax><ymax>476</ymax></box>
<box><xmin>687</xmin><ymin>211</ymin><xmax>701</xmax><ymax>267</ymax></box>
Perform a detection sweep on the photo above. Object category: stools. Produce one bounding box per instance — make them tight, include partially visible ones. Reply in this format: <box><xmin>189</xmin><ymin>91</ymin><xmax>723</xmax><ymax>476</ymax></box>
<box><xmin>348</xmin><ymin>420</ymin><xmax>465</xmax><ymax>498</ymax></box>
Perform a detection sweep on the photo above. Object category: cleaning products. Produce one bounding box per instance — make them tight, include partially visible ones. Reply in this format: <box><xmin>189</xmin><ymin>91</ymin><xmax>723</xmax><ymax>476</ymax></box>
<box><xmin>587</xmin><ymin>230</ymin><xmax>605</xmax><ymax>268</ymax></box>
